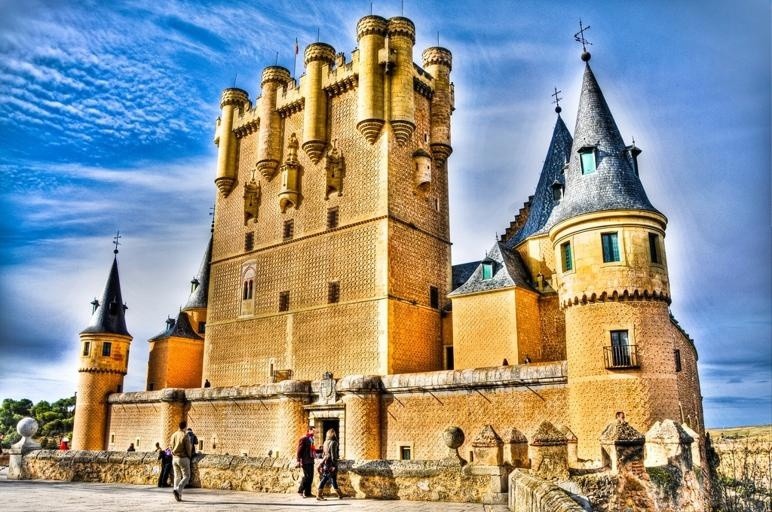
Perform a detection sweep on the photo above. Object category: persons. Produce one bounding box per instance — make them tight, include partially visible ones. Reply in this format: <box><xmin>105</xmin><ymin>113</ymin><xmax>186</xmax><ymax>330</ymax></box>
<box><xmin>158</xmin><ymin>446</ymin><xmax>172</xmax><ymax>488</ymax></box>
<box><xmin>524</xmin><ymin>352</ymin><xmax>529</xmax><ymax>365</ymax></box>
<box><xmin>296</xmin><ymin>427</ymin><xmax>317</xmax><ymax>499</ymax></box>
<box><xmin>317</xmin><ymin>428</ymin><xmax>346</xmax><ymax>500</ymax></box>
<box><xmin>204</xmin><ymin>379</ymin><xmax>212</xmax><ymax>389</ymax></box>
<box><xmin>185</xmin><ymin>428</ymin><xmax>198</xmax><ymax>488</ymax></box>
<box><xmin>127</xmin><ymin>443</ymin><xmax>135</xmax><ymax>451</ymax></box>
<box><xmin>170</xmin><ymin>420</ymin><xmax>191</xmax><ymax>502</ymax></box>
<box><xmin>0</xmin><ymin>434</ymin><xmax>4</xmax><ymax>454</ymax></box>
<box><xmin>502</xmin><ymin>357</ymin><xmax>509</xmax><ymax>366</ymax></box>
<box><xmin>154</xmin><ymin>442</ymin><xmax>161</xmax><ymax>452</ymax></box>
<box><xmin>615</xmin><ymin>411</ymin><xmax>626</xmax><ymax>420</ymax></box>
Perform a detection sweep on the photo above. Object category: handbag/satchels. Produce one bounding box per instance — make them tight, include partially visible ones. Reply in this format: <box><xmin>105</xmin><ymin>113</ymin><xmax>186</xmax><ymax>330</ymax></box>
<box><xmin>317</xmin><ymin>454</ymin><xmax>335</xmax><ymax>477</ymax></box>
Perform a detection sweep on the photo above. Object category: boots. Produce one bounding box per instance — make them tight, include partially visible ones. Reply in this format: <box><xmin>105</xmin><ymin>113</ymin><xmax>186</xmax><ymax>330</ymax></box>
<box><xmin>334</xmin><ymin>489</ymin><xmax>349</xmax><ymax>499</ymax></box>
<box><xmin>317</xmin><ymin>489</ymin><xmax>327</xmax><ymax>501</ymax></box>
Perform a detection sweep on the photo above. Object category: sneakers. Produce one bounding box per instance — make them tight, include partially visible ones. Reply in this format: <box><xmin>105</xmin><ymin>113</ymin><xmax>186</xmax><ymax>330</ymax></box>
<box><xmin>174</xmin><ymin>490</ymin><xmax>182</xmax><ymax>501</ymax></box>
